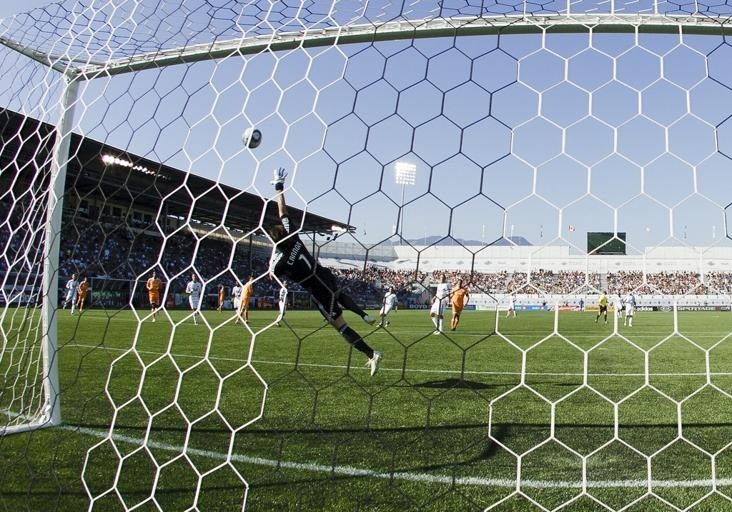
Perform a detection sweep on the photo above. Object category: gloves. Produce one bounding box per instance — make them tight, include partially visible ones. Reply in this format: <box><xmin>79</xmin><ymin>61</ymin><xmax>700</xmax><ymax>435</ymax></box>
<box><xmin>270</xmin><ymin>168</ymin><xmax>288</xmax><ymax>190</ymax></box>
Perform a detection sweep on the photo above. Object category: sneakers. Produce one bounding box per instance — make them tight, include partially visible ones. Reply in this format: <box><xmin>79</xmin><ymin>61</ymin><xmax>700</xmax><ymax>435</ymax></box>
<box><xmin>433</xmin><ymin>330</ymin><xmax>441</xmax><ymax>335</ymax></box>
<box><xmin>376</xmin><ymin>323</ymin><xmax>383</xmax><ymax>327</ymax></box>
<box><xmin>385</xmin><ymin>322</ymin><xmax>390</xmax><ymax>327</ymax></box>
<box><xmin>364</xmin><ymin>315</ymin><xmax>376</xmax><ymax>325</ymax></box>
<box><xmin>367</xmin><ymin>351</ymin><xmax>384</xmax><ymax>376</ymax></box>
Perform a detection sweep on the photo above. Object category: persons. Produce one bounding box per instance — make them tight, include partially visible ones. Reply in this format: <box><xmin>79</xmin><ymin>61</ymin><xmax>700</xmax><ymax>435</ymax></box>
<box><xmin>1</xmin><ymin>203</ymin><xmax>322</xmax><ymax>312</ymax></box>
<box><xmin>267</xmin><ymin>166</ymin><xmax>385</xmax><ymax>377</ymax></box>
<box><xmin>429</xmin><ymin>273</ymin><xmax>451</xmax><ymax>335</ymax></box>
<box><xmin>61</xmin><ymin>273</ymin><xmax>79</xmax><ymax>315</ymax></box>
<box><xmin>375</xmin><ymin>286</ymin><xmax>399</xmax><ymax>328</ymax></box>
<box><xmin>275</xmin><ymin>278</ymin><xmax>290</xmax><ymax>329</ymax></box>
<box><xmin>613</xmin><ymin>292</ymin><xmax>624</xmax><ymax>318</ymax></box>
<box><xmin>145</xmin><ymin>271</ymin><xmax>162</xmax><ymax>322</ymax></box>
<box><xmin>622</xmin><ymin>293</ymin><xmax>637</xmax><ymax>327</ymax></box>
<box><xmin>449</xmin><ymin>279</ymin><xmax>470</xmax><ymax>330</ymax></box>
<box><xmin>234</xmin><ymin>271</ymin><xmax>254</xmax><ymax>325</ymax></box>
<box><xmin>184</xmin><ymin>273</ymin><xmax>202</xmax><ymax>326</ymax></box>
<box><xmin>335</xmin><ymin>267</ymin><xmax>731</xmax><ymax>312</ymax></box>
<box><xmin>594</xmin><ymin>290</ymin><xmax>612</xmax><ymax>324</ymax></box>
<box><xmin>504</xmin><ymin>289</ymin><xmax>519</xmax><ymax>318</ymax></box>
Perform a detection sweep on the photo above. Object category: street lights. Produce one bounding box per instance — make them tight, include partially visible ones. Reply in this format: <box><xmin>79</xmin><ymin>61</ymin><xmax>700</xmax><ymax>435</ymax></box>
<box><xmin>395</xmin><ymin>161</ymin><xmax>416</xmax><ymax>245</ymax></box>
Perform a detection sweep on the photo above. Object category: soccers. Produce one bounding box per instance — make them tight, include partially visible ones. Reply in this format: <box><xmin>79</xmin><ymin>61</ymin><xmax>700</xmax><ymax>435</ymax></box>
<box><xmin>242</xmin><ymin>128</ymin><xmax>262</xmax><ymax>148</ymax></box>
